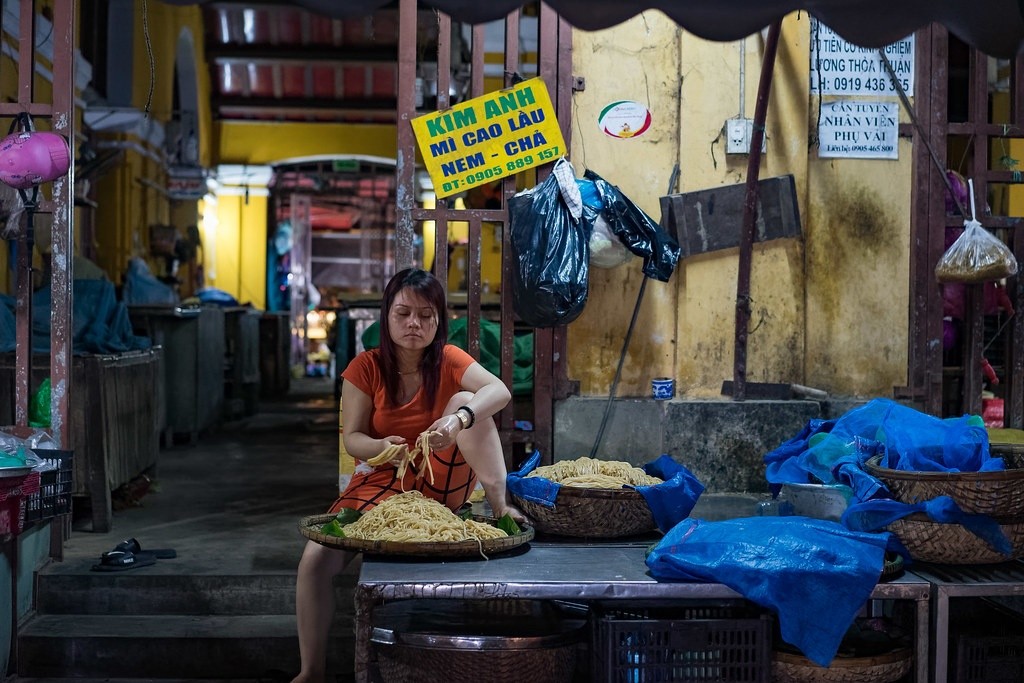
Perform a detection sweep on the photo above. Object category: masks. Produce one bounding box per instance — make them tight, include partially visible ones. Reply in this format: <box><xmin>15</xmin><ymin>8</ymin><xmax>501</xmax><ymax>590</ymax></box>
<box><xmin>552</xmin><ymin>156</ymin><xmax>583</xmax><ymax>226</ymax></box>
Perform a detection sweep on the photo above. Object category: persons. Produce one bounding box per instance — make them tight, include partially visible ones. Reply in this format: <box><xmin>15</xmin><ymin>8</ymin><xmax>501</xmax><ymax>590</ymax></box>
<box><xmin>292</xmin><ymin>268</ymin><xmax>533</xmax><ymax>683</ymax></box>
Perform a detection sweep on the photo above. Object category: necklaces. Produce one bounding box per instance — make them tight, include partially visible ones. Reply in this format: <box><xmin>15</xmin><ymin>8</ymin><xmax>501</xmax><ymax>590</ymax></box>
<box><xmin>399</xmin><ymin>370</ymin><xmax>417</xmax><ymax>375</ymax></box>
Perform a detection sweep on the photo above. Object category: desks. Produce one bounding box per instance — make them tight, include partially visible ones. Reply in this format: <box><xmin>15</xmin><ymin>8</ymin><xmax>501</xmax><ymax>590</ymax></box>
<box><xmin>907</xmin><ymin>559</ymin><xmax>1023</xmax><ymax>682</ymax></box>
<box><xmin>354</xmin><ymin>530</ymin><xmax>931</xmax><ymax>682</ymax></box>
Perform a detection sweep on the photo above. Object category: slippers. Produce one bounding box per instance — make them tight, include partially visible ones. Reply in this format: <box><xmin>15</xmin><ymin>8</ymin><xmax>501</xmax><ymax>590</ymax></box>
<box><xmin>91</xmin><ymin>538</ymin><xmax>178</xmax><ymax>571</ymax></box>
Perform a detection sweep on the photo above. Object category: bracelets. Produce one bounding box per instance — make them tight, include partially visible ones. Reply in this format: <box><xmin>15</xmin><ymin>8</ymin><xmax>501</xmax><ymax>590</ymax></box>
<box><xmin>458</xmin><ymin>406</ymin><xmax>475</xmax><ymax>429</ymax></box>
<box><xmin>453</xmin><ymin>412</ymin><xmax>468</xmax><ymax>429</ymax></box>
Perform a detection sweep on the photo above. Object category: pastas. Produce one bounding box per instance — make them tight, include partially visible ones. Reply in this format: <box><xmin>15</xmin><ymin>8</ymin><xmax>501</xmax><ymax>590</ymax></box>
<box><xmin>339</xmin><ymin>490</ymin><xmax>509</xmax><ymax>564</ymax></box>
<box><xmin>521</xmin><ymin>455</ymin><xmax>667</xmax><ymax>490</ymax></box>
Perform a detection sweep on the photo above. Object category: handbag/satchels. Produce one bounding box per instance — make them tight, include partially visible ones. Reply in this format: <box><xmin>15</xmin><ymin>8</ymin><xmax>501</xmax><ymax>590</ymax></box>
<box><xmin>934</xmin><ymin>177</ymin><xmax>1019</xmax><ymax>287</ymax></box>
<box><xmin>506</xmin><ymin>154</ymin><xmax>591</xmax><ymax>329</ymax></box>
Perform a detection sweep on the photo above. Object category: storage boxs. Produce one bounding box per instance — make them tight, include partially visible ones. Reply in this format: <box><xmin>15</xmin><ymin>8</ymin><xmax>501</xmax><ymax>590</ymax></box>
<box><xmin>22</xmin><ymin>449</ymin><xmax>76</xmax><ymax>524</ymax></box>
<box><xmin>587</xmin><ymin>595</ymin><xmax>771</xmax><ymax>682</ymax></box>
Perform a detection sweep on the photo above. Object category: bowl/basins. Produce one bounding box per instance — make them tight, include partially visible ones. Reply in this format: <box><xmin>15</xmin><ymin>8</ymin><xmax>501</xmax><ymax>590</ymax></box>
<box><xmin>782</xmin><ymin>481</ymin><xmax>853</xmax><ymax>521</ymax></box>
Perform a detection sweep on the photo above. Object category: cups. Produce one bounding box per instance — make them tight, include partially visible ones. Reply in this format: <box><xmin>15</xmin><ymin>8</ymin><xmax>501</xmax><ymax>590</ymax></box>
<box><xmin>652</xmin><ymin>377</ymin><xmax>674</xmax><ymax>401</ymax></box>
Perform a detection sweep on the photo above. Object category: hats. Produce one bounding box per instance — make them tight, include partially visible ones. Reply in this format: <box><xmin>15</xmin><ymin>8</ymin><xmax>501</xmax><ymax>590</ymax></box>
<box><xmin>0</xmin><ymin>131</ymin><xmax>70</xmax><ymax>189</ymax></box>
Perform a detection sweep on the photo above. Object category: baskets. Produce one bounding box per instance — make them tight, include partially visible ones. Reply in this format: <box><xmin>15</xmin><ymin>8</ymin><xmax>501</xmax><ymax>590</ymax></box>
<box><xmin>0</xmin><ymin>448</ymin><xmax>74</xmax><ymax>536</ymax></box>
<box><xmin>863</xmin><ymin>443</ymin><xmax>1024</xmax><ymax>566</ymax></box>
<box><xmin>769</xmin><ymin>614</ymin><xmax>916</xmax><ymax>683</ymax></box>
<box><xmin>507</xmin><ymin>471</ymin><xmax>681</xmax><ymax>539</ymax></box>
<box><xmin>586</xmin><ymin>604</ymin><xmax>768</xmax><ymax>683</ymax></box>
<box><xmin>370</xmin><ymin>598</ymin><xmax>589</xmax><ymax>683</ymax></box>
<box><xmin>893</xmin><ymin>593</ymin><xmax>1024</xmax><ymax>683</ymax></box>
